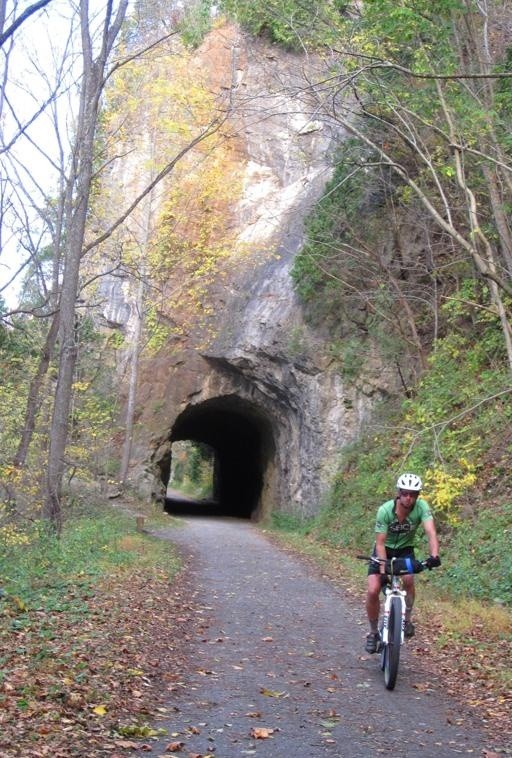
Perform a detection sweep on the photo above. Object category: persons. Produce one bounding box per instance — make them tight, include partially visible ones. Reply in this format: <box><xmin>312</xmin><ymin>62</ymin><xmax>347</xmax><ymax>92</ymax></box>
<box><xmin>365</xmin><ymin>472</ymin><xmax>440</xmax><ymax>653</ymax></box>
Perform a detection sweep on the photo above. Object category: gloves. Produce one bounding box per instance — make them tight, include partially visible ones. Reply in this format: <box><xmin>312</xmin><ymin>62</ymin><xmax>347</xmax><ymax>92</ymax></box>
<box><xmin>381</xmin><ymin>574</ymin><xmax>388</xmax><ymax>595</ymax></box>
<box><xmin>427</xmin><ymin>556</ymin><xmax>442</xmax><ymax>568</ymax></box>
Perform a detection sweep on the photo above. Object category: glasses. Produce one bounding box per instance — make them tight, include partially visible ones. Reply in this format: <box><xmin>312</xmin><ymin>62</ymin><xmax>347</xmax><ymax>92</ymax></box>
<box><xmin>398</xmin><ymin>490</ymin><xmax>420</xmax><ymax>496</ymax></box>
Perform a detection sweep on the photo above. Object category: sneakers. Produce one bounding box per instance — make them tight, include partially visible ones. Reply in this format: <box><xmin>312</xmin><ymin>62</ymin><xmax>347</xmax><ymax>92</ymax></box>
<box><xmin>405</xmin><ymin>622</ymin><xmax>415</xmax><ymax>636</ymax></box>
<box><xmin>366</xmin><ymin>629</ymin><xmax>381</xmax><ymax>651</ymax></box>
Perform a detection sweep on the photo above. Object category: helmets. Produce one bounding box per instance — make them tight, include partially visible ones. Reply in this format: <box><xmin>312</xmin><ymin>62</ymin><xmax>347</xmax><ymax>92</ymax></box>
<box><xmin>397</xmin><ymin>474</ymin><xmax>422</xmax><ymax>490</ymax></box>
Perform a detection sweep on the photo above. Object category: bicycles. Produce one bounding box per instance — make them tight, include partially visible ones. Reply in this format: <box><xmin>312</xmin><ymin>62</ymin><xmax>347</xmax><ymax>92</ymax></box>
<box><xmin>357</xmin><ymin>555</ymin><xmax>441</xmax><ymax>691</ymax></box>
